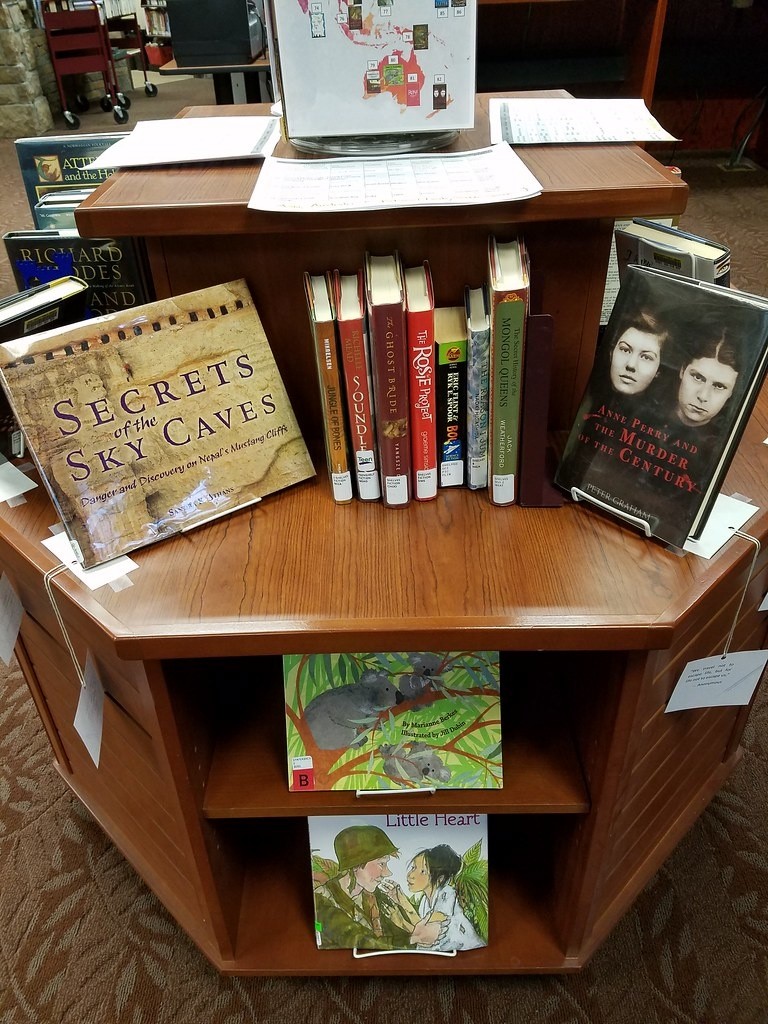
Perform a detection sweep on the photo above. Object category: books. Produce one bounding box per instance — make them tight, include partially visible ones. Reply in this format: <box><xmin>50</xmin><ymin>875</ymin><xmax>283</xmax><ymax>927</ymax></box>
<box><xmin>32</xmin><ymin>0</ymin><xmax>171</xmax><ymax>60</ymax></box>
<box><xmin>0</xmin><ymin>129</ymin><xmax>768</xmax><ymax>573</ymax></box>
<box><xmin>283</xmin><ymin>651</ymin><xmax>503</xmax><ymax>792</ymax></box>
<box><xmin>308</xmin><ymin>814</ymin><xmax>489</xmax><ymax>951</ymax></box>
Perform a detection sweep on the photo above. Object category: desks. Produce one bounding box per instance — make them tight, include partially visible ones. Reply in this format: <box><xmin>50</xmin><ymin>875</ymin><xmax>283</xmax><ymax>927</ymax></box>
<box><xmin>159</xmin><ymin>49</ymin><xmax>271</xmax><ymax>104</ymax></box>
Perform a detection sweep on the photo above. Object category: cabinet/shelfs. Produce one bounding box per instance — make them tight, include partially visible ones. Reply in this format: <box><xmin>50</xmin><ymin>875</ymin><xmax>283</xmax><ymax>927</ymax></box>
<box><xmin>39</xmin><ymin>0</ymin><xmax>159</xmax><ymax>128</ymax></box>
<box><xmin>0</xmin><ymin>89</ymin><xmax>768</xmax><ymax>978</ymax></box>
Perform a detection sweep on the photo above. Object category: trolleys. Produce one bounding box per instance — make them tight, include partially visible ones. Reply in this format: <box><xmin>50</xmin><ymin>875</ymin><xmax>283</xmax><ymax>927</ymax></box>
<box><xmin>41</xmin><ymin>0</ymin><xmax>159</xmax><ymax>131</ymax></box>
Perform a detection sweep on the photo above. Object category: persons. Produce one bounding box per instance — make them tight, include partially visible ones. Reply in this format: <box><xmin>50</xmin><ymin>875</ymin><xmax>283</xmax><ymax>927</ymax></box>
<box><xmin>667</xmin><ymin>326</ymin><xmax>742</xmax><ymax>432</ymax></box>
<box><xmin>590</xmin><ymin>308</ymin><xmax>670</xmax><ymax>405</ymax></box>
